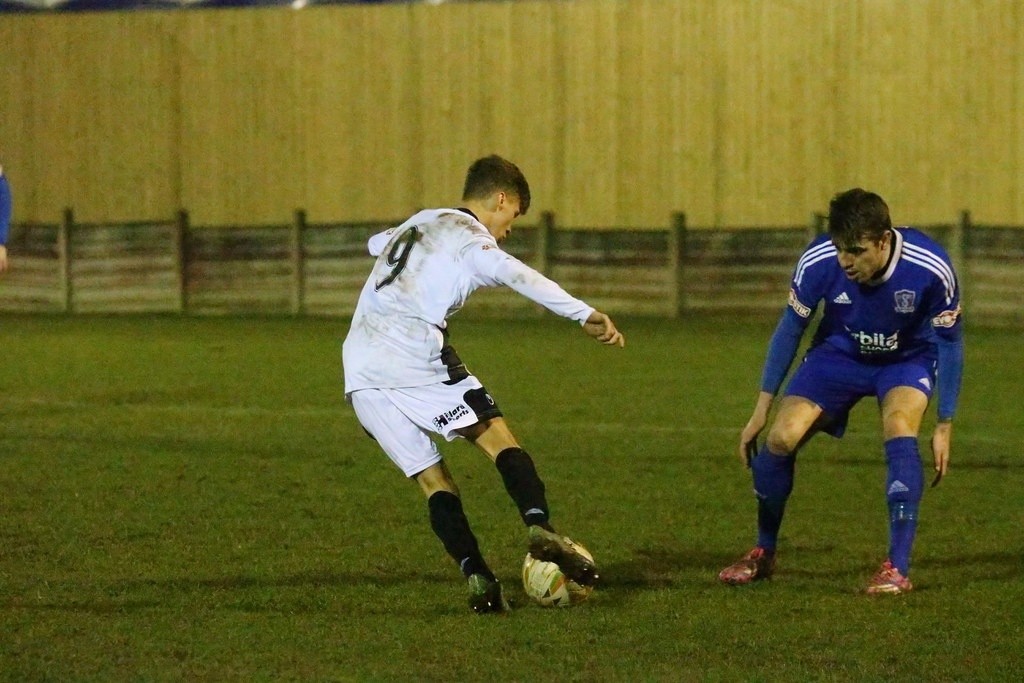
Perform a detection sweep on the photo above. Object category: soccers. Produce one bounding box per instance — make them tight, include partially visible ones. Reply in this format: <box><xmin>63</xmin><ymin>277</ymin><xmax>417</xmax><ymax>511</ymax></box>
<box><xmin>522</xmin><ymin>536</ymin><xmax>595</xmax><ymax>608</ymax></box>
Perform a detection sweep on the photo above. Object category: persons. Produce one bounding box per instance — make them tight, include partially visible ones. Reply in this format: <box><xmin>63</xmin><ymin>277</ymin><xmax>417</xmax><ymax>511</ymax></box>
<box><xmin>342</xmin><ymin>154</ymin><xmax>625</xmax><ymax>615</ymax></box>
<box><xmin>718</xmin><ymin>188</ymin><xmax>963</xmax><ymax>594</ymax></box>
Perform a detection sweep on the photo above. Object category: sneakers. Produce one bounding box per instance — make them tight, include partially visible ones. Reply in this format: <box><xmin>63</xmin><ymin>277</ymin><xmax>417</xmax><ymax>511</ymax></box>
<box><xmin>527</xmin><ymin>525</ymin><xmax>602</xmax><ymax>586</ymax></box>
<box><xmin>467</xmin><ymin>574</ymin><xmax>502</xmax><ymax>611</ymax></box>
<box><xmin>720</xmin><ymin>547</ymin><xmax>775</xmax><ymax>586</ymax></box>
<box><xmin>864</xmin><ymin>561</ymin><xmax>912</xmax><ymax>595</ymax></box>
